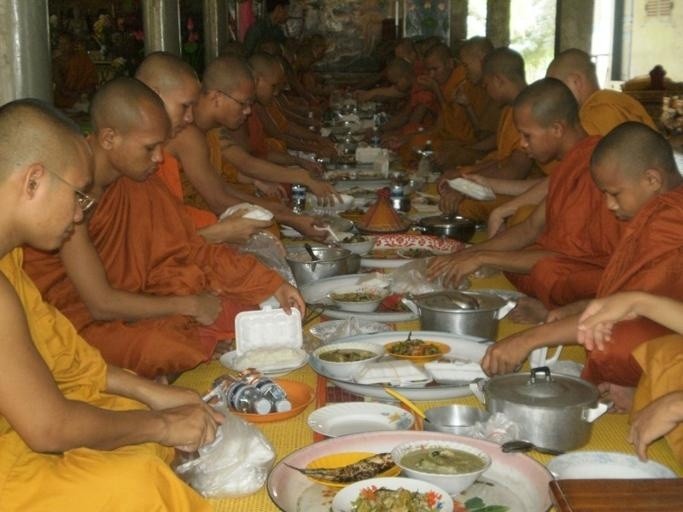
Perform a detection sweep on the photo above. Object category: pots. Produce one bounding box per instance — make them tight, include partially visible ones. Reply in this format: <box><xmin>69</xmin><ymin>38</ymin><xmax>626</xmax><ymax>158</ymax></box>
<box><xmin>409</xmin><ymin>213</ymin><xmax>485</xmax><ymax>242</ymax></box>
<box><xmin>400</xmin><ymin>291</ymin><xmax>520</xmax><ymax>339</ymax></box>
<box><xmin>286</xmin><ymin>247</ymin><xmax>360</xmax><ymax>286</ymax></box>
<box><xmin>470</xmin><ymin>368</ymin><xmax>614</xmax><ymax>453</ymax></box>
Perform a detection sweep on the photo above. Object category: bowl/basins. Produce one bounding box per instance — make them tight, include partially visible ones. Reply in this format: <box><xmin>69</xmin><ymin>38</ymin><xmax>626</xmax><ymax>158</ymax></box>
<box><xmin>422</xmin><ymin>404</ymin><xmax>492</xmax><ymax>434</ymax></box>
<box><xmin>390</xmin><ymin>437</ymin><xmax>493</xmax><ymax>497</ymax></box>
<box><xmin>326</xmin><ymin>285</ymin><xmax>387</xmax><ymax>313</ymax></box>
<box><xmin>332</xmin><ymin>475</ymin><xmax>454</xmax><ymax>512</ymax></box>
<box><xmin>307</xmin><ymin>329</ymin><xmax>500</xmax><ymax>399</ymax></box>
<box><xmin>313</xmin><ymin>342</ymin><xmax>383</xmax><ymax>372</ymax></box>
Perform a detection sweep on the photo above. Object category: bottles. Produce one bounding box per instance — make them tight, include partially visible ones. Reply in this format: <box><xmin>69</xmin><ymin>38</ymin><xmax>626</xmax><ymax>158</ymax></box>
<box><xmin>242</xmin><ymin>367</ymin><xmax>291</xmax><ymax>415</ymax></box>
<box><xmin>280</xmin><ymin>101</ymin><xmax>434</xmax><ymax>269</ymax></box>
<box><xmin>213</xmin><ymin>374</ymin><xmax>270</xmax><ymax>415</ymax></box>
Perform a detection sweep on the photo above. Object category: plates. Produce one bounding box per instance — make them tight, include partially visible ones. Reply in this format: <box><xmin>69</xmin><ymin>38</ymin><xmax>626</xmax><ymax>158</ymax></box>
<box><xmin>265</xmin><ymin>429</ymin><xmax>557</xmax><ymax>512</ymax></box>
<box><xmin>219</xmin><ymin>348</ymin><xmax>311</xmax><ymax>374</ymax></box>
<box><xmin>309</xmin><ymin>320</ymin><xmax>392</xmax><ymax>337</ymax></box>
<box><xmin>306</xmin><ymin>450</ymin><xmax>399</xmax><ymax>488</ymax></box>
<box><xmin>297</xmin><ymin>273</ymin><xmax>431</xmax><ymax>320</ymax></box>
<box><xmin>340</xmin><ymin>233</ymin><xmax>469</xmax><ymax>268</ymax></box>
<box><xmin>306</xmin><ymin>400</ymin><xmax>415</xmax><ymax>436</ymax></box>
<box><xmin>386</xmin><ymin>339</ymin><xmax>449</xmax><ymax>363</ymax></box>
<box><xmin>219</xmin><ymin>378</ymin><xmax>316</xmax><ymax>423</ymax></box>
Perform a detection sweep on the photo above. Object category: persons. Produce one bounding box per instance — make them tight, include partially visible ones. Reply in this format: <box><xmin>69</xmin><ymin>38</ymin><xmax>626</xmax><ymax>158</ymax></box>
<box><xmin>357</xmin><ymin>34</ymin><xmax>659</xmax><ymax>238</ymax></box>
<box><xmin>22</xmin><ymin>76</ymin><xmax>305</xmax><ymax>379</ymax></box>
<box><xmin>1</xmin><ymin>96</ymin><xmax>224</xmax><ymax>508</ymax></box>
<box><xmin>576</xmin><ymin>291</ymin><xmax>682</xmax><ymax>464</ymax></box>
<box><xmin>172</xmin><ymin>3</ymin><xmax>348</xmax><ymax>242</ymax></box>
<box><xmin>482</xmin><ymin>121</ymin><xmax>683</xmax><ymax>413</ymax></box>
<box><xmin>425</xmin><ymin>77</ymin><xmax>634</xmax><ymax>323</ymax></box>
<box><xmin>137</xmin><ymin>52</ymin><xmax>280</xmax><ymax>252</ymax></box>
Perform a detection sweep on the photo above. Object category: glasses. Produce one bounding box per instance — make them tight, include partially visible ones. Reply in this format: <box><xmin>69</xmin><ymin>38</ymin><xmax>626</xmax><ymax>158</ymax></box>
<box><xmin>218</xmin><ymin>89</ymin><xmax>256</xmax><ymax>109</ymax></box>
<box><xmin>30</xmin><ymin>162</ymin><xmax>95</xmax><ymax>212</ymax></box>
